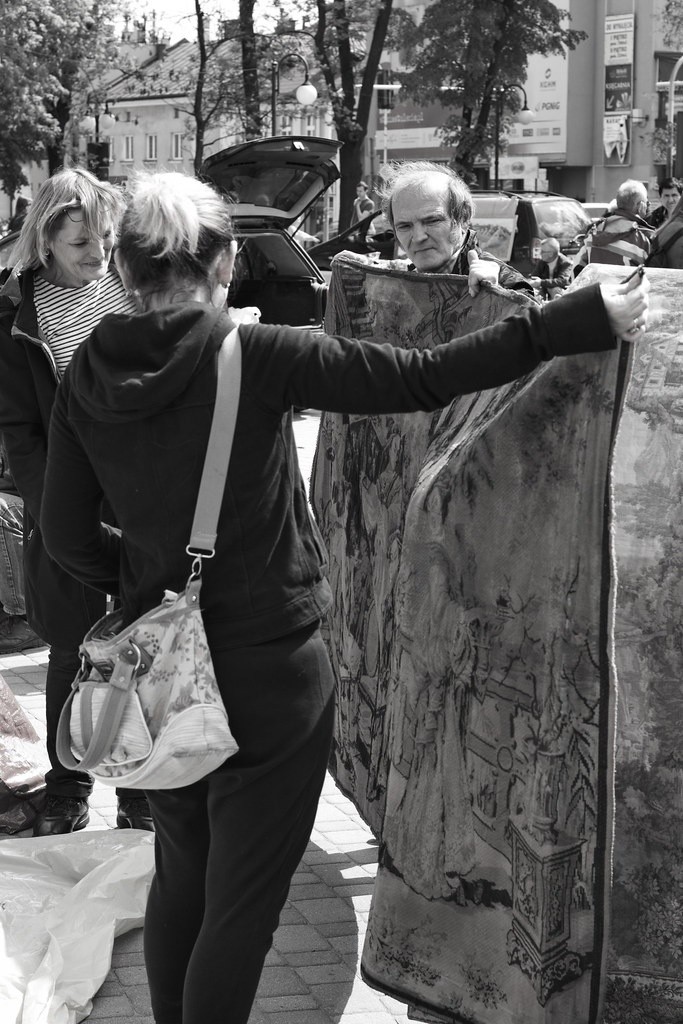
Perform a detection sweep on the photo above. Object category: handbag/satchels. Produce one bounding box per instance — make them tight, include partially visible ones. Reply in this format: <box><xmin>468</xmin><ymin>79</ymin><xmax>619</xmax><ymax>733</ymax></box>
<box><xmin>59</xmin><ymin>589</ymin><xmax>244</xmax><ymax>792</ymax></box>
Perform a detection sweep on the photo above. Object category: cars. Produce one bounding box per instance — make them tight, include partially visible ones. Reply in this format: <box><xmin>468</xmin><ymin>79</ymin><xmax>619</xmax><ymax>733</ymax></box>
<box><xmin>0</xmin><ymin>134</ymin><xmax>345</xmax><ymax>339</ymax></box>
<box><xmin>309</xmin><ymin>186</ymin><xmax>594</xmax><ymax>283</ymax></box>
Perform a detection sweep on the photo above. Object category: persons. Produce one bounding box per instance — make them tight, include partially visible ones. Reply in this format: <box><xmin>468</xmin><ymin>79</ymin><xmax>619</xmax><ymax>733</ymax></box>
<box><xmin>0</xmin><ymin>165</ymin><xmax>183</xmax><ymax>836</ymax></box>
<box><xmin>575</xmin><ymin>177</ymin><xmax>658</xmax><ymax>269</ymax></box>
<box><xmin>36</xmin><ymin>167</ymin><xmax>652</xmax><ymax>1023</ymax></box>
<box><xmin>352</xmin><ymin>179</ymin><xmax>376</xmax><ymax>241</ymax></box>
<box><xmin>6</xmin><ymin>194</ymin><xmax>35</xmax><ymax>233</ymax></box>
<box><xmin>529</xmin><ymin>237</ymin><xmax>573</xmax><ymax>299</ymax></box>
<box><xmin>643</xmin><ymin>175</ymin><xmax>683</xmax><ymax>231</ymax></box>
<box><xmin>335</xmin><ymin>160</ymin><xmax>539</xmax><ymax>306</ymax></box>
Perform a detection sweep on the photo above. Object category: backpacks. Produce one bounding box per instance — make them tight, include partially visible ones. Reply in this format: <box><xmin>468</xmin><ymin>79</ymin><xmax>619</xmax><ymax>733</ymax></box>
<box><xmin>644</xmin><ymin>228</ymin><xmax>682</xmax><ymax>268</ymax></box>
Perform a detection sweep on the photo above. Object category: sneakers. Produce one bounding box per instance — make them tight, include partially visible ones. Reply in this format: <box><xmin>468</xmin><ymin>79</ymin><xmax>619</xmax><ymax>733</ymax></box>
<box><xmin>2</xmin><ymin>617</ymin><xmax>46</xmax><ymax>653</ymax></box>
<box><xmin>34</xmin><ymin>798</ymin><xmax>88</xmax><ymax>836</ymax></box>
<box><xmin>117</xmin><ymin>798</ymin><xmax>155</xmax><ymax>833</ymax></box>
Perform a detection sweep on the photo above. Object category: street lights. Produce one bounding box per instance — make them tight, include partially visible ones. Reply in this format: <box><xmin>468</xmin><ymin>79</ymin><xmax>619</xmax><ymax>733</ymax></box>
<box><xmin>493</xmin><ymin>84</ymin><xmax>534</xmax><ymax>193</ymax></box>
<box><xmin>268</xmin><ymin>54</ymin><xmax>317</xmax><ymax>137</ymax></box>
